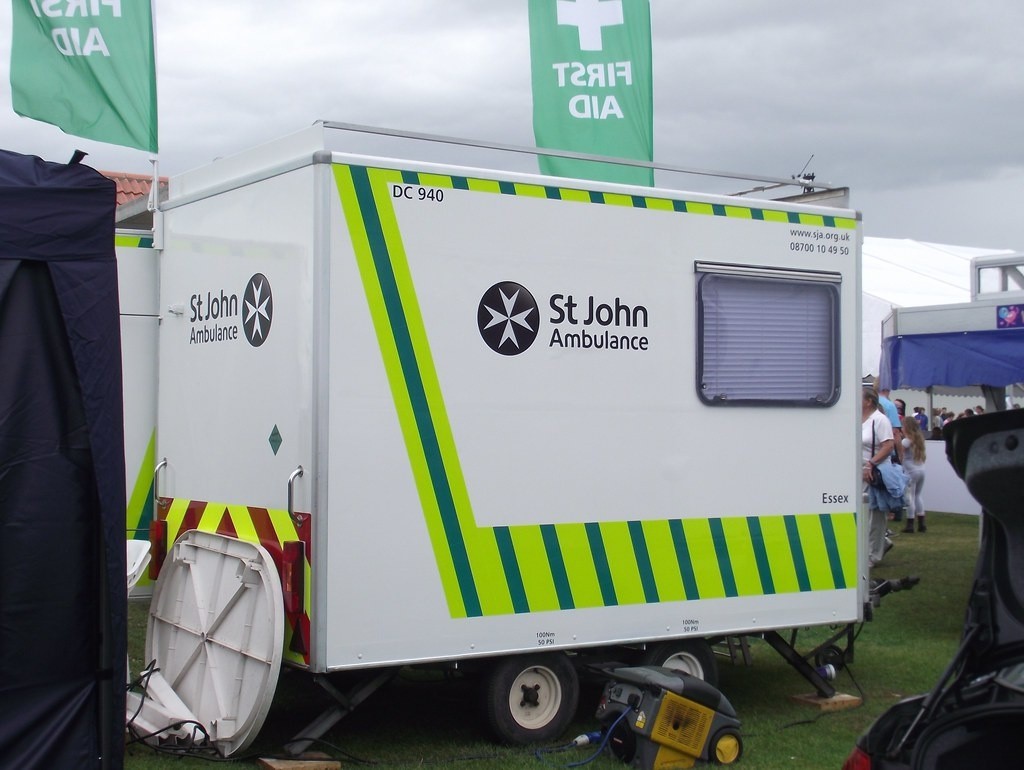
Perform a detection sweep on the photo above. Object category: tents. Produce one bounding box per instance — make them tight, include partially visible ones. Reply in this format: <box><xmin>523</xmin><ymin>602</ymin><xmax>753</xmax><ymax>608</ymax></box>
<box><xmin>0</xmin><ymin>150</ymin><xmax>127</xmax><ymax>770</ymax></box>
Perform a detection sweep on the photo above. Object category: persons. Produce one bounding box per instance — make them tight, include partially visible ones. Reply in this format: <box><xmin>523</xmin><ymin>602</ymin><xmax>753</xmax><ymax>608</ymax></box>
<box><xmin>932</xmin><ymin>407</ymin><xmax>955</xmax><ymax>430</ymax></box>
<box><xmin>1011</xmin><ymin>403</ymin><xmax>1020</xmax><ymax>409</ymax></box>
<box><xmin>912</xmin><ymin>407</ymin><xmax>929</xmax><ymax>431</ymax></box>
<box><xmin>872</xmin><ymin>375</ymin><xmax>906</xmax><ymax>522</ymax></box>
<box><xmin>925</xmin><ymin>427</ymin><xmax>945</xmax><ymax>440</ymax></box>
<box><xmin>862</xmin><ymin>388</ymin><xmax>894</xmax><ymax>569</ymax></box>
<box><xmin>957</xmin><ymin>406</ymin><xmax>984</xmax><ymax>420</ymax></box>
<box><xmin>901</xmin><ymin>417</ymin><xmax>928</xmax><ymax>534</ymax></box>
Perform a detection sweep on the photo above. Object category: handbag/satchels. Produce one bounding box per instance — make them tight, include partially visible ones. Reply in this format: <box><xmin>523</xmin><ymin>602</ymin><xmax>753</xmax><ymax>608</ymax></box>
<box><xmin>870</xmin><ymin>461</ymin><xmax>901</xmax><ymax>491</ymax></box>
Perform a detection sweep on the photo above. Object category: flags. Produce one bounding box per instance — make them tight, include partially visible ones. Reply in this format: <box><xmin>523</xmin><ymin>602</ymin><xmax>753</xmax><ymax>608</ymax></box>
<box><xmin>10</xmin><ymin>0</ymin><xmax>158</xmax><ymax>157</ymax></box>
<box><xmin>529</xmin><ymin>0</ymin><xmax>656</xmax><ymax>186</ymax></box>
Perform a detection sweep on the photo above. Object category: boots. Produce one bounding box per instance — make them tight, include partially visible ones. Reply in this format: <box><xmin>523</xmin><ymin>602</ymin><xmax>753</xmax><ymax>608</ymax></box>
<box><xmin>917</xmin><ymin>515</ymin><xmax>926</xmax><ymax>532</ymax></box>
<box><xmin>901</xmin><ymin>518</ymin><xmax>914</xmax><ymax>533</ymax></box>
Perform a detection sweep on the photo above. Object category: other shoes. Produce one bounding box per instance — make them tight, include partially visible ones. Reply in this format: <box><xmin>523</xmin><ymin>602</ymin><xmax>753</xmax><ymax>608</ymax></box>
<box><xmin>884</xmin><ymin>537</ymin><xmax>893</xmax><ymax>552</ymax></box>
<box><xmin>893</xmin><ymin>513</ymin><xmax>902</xmax><ymax>521</ymax></box>
